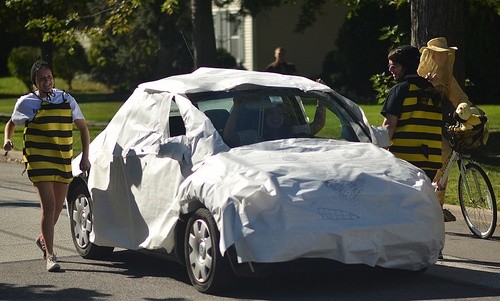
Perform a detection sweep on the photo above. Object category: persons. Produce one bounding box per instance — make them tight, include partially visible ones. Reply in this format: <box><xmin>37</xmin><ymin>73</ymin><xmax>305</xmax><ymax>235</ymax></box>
<box><xmin>417</xmin><ymin>37</ymin><xmax>486</xmax><ymax>222</ymax></box>
<box><xmin>264</xmin><ymin>48</ymin><xmax>300</xmax><ymax>77</ymax></box>
<box><xmin>3</xmin><ymin>62</ymin><xmax>92</xmax><ymax>273</ymax></box>
<box><xmin>380</xmin><ymin>45</ymin><xmax>444</xmax><ymax>261</ymax></box>
<box><xmin>223</xmin><ymin>77</ymin><xmax>326</xmax><ymax>147</ymax></box>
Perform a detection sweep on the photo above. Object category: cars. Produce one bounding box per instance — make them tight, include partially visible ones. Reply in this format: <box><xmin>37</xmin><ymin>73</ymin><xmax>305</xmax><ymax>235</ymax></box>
<box><xmin>67</xmin><ymin>67</ymin><xmax>446</xmax><ymax>292</ymax></box>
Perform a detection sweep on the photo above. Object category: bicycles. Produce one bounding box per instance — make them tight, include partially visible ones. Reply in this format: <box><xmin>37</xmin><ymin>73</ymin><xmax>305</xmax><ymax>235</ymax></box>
<box><xmin>433</xmin><ymin>113</ymin><xmax>498</xmax><ymax>239</ymax></box>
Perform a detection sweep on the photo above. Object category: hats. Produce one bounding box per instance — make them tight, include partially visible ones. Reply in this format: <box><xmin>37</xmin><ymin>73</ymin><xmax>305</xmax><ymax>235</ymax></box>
<box><xmin>418</xmin><ymin>38</ymin><xmax>458</xmax><ymax>54</ymax></box>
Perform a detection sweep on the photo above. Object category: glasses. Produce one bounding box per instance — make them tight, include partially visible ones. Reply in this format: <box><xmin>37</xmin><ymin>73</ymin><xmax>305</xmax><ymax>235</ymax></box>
<box><xmin>389</xmin><ymin>64</ymin><xmax>400</xmax><ymax>70</ymax></box>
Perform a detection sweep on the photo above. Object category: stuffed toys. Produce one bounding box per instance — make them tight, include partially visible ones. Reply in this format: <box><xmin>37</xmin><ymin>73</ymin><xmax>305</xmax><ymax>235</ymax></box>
<box><xmin>448</xmin><ymin>102</ymin><xmax>489</xmax><ymax>145</ymax></box>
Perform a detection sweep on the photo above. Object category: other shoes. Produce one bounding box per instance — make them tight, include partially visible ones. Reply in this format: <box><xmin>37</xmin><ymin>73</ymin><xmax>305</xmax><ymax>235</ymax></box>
<box><xmin>442</xmin><ymin>208</ymin><xmax>456</xmax><ymax>223</ymax></box>
<box><xmin>46</xmin><ymin>253</ymin><xmax>60</xmax><ymax>271</ymax></box>
<box><xmin>36</xmin><ymin>236</ymin><xmax>46</xmax><ymax>260</ymax></box>
<box><xmin>437</xmin><ymin>250</ymin><xmax>444</xmax><ymax>261</ymax></box>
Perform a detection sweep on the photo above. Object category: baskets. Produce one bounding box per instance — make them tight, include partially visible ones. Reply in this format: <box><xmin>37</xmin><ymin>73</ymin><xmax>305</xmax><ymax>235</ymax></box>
<box><xmin>445</xmin><ymin>123</ymin><xmax>485</xmax><ymax>155</ymax></box>
